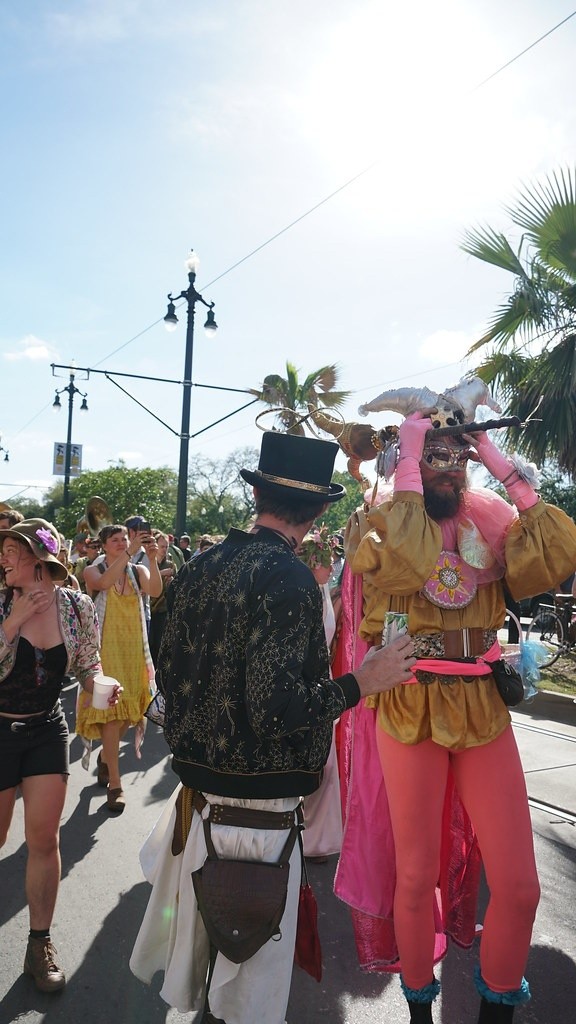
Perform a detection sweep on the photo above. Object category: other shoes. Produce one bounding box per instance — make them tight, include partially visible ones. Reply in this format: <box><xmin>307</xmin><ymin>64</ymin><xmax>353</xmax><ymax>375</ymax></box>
<box><xmin>107</xmin><ymin>782</ymin><xmax>126</xmax><ymax>812</ymax></box>
<box><xmin>96</xmin><ymin>750</ymin><xmax>110</xmax><ymax>787</ymax></box>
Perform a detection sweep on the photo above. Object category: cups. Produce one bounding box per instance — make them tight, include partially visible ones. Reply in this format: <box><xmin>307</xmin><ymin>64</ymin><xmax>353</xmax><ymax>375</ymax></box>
<box><xmin>92</xmin><ymin>676</ymin><xmax>117</xmax><ymax>711</ymax></box>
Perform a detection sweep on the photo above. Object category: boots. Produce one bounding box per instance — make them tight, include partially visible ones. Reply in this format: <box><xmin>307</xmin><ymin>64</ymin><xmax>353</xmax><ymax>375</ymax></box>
<box><xmin>399</xmin><ymin>972</ymin><xmax>440</xmax><ymax>1024</ymax></box>
<box><xmin>474</xmin><ymin>963</ymin><xmax>532</xmax><ymax>1024</ymax></box>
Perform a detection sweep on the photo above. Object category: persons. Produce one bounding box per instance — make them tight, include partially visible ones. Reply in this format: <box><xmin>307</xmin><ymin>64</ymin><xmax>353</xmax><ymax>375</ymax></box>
<box><xmin>128</xmin><ymin>432</ymin><xmax>418</xmax><ymax>1024</ymax></box>
<box><xmin>341</xmin><ymin>377</ymin><xmax>576</xmax><ymax>1024</ymax></box>
<box><xmin>0</xmin><ymin>517</ymin><xmax>124</xmax><ymax>992</ymax></box>
<box><xmin>0</xmin><ymin>501</ymin><xmax>346</xmax><ymax>671</ymax></box>
<box><xmin>75</xmin><ymin>524</ymin><xmax>164</xmax><ymax>812</ymax></box>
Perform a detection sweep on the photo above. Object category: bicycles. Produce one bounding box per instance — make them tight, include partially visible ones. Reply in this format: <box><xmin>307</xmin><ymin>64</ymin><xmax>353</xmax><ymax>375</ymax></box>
<box><xmin>526</xmin><ymin>593</ymin><xmax>576</xmax><ymax>669</ymax></box>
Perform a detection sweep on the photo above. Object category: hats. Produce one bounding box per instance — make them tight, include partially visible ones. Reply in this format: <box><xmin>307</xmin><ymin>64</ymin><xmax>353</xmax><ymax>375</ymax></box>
<box><xmin>0</xmin><ymin>518</ymin><xmax>68</xmax><ymax>581</ymax></box>
<box><xmin>240</xmin><ymin>431</ymin><xmax>347</xmax><ymax>500</ymax></box>
<box><xmin>358</xmin><ymin>373</ymin><xmax>502</xmax><ymax>455</ymax></box>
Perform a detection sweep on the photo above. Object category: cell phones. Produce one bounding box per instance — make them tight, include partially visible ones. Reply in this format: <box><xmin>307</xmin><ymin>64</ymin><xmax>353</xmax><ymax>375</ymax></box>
<box><xmin>139</xmin><ymin>520</ymin><xmax>152</xmax><ymax>544</ymax></box>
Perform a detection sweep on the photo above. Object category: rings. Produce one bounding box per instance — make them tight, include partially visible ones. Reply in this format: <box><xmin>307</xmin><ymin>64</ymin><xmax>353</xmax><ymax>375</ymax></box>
<box><xmin>141</xmin><ymin>539</ymin><xmax>143</xmax><ymax>542</ymax></box>
<box><xmin>27</xmin><ymin>592</ymin><xmax>33</xmax><ymax>598</ymax></box>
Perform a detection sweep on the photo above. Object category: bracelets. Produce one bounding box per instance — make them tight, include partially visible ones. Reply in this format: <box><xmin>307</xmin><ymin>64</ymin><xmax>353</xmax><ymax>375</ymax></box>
<box><xmin>125</xmin><ymin>548</ymin><xmax>132</xmax><ymax>559</ymax></box>
<box><xmin>501</xmin><ymin>447</ymin><xmax>542</xmax><ymax>488</ymax></box>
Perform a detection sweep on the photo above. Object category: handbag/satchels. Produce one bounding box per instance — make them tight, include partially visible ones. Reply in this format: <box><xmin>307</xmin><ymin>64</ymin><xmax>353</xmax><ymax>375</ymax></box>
<box><xmin>191</xmin><ymin>791</ymin><xmax>303</xmax><ymax>965</ymax></box>
<box><xmin>296</xmin><ymin>883</ymin><xmax>323</xmax><ymax>983</ymax></box>
<box><xmin>488</xmin><ymin>659</ymin><xmax>524</xmax><ymax>706</ymax></box>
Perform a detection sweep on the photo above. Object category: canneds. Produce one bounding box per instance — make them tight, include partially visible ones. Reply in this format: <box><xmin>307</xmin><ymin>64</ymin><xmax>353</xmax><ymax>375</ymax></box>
<box><xmin>381</xmin><ymin>611</ymin><xmax>409</xmax><ymax>647</ymax></box>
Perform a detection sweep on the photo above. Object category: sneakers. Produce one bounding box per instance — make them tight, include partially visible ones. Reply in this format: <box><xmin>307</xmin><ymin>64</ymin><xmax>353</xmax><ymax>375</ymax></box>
<box><xmin>24</xmin><ymin>937</ymin><xmax>65</xmax><ymax>992</ymax></box>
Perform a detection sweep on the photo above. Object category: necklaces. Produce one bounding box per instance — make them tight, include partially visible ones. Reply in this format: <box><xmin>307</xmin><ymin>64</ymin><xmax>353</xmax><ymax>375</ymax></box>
<box><xmin>35</xmin><ymin>594</ymin><xmax>55</xmax><ymax>614</ymax></box>
<box><xmin>118</xmin><ymin>577</ymin><xmax>122</xmax><ymax>585</ymax></box>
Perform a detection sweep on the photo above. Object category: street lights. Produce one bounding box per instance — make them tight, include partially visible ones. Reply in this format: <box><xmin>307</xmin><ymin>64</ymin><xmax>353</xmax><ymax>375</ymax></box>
<box><xmin>219</xmin><ymin>505</ymin><xmax>224</xmax><ymax>535</ymax></box>
<box><xmin>186</xmin><ymin>509</ymin><xmax>191</xmax><ymax>534</ymax></box>
<box><xmin>51</xmin><ymin>356</ymin><xmax>90</xmax><ymax>509</ymax></box>
<box><xmin>163</xmin><ymin>248</ymin><xmax>219</xmax><ymax>536</ymax></box>
<box><xmin>201</xmin><ymin>507</ymin><xmax>207</xmax><ymax>535</ymax></box>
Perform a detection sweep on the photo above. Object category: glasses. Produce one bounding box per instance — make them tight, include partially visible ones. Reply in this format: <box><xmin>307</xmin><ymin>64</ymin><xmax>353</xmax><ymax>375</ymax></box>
<box><xmin>85</xmin><ymin>544</ymin><xmax>101</xmax><ymax>549</ymax></box>
<box><xmin>131</xmin><ymin>526</ymin><xmax>138</xmax><ymax>531</ymax></box>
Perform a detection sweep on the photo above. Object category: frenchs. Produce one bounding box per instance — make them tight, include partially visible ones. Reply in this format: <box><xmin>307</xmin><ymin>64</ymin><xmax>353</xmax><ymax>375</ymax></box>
<box><xmin>78</xmin><ymin>496</ymin><xmax>112</xmax><ymax>537</ymax></box>
